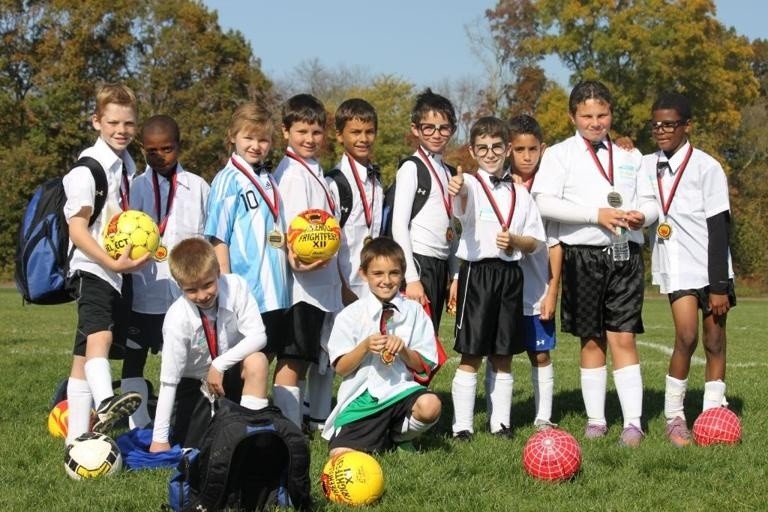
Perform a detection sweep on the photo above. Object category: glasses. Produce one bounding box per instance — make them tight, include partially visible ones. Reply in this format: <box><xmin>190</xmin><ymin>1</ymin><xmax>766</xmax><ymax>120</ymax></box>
<box><xmin>648</xmin><ymin>117</ymin><xmax>689</xmax><ymax>134</ymax></box>
<box><xmin>412</xmin><ymin>120</ymin><xmax>455</xmax><ymax>139</ymax></box>
<box><xmin>470</xmin><ymin>140</ymin><xmax>510</xmax><ymax>159</ymax></box>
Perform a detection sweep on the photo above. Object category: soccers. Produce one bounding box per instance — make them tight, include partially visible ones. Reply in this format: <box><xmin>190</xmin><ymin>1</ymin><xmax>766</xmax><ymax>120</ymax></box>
<box><xmin>524</xmin><ymin>428</ymin><xmax>582</xmax><ymax>482</ymax></box>
<box><xmin>46</xmin><ymin>400</ymin><xmax>95</xmax><ymax>437</ymax></box>
<box><xmin>321</xmin><ymin>452</ymin><xmax>385</xmax><ymax>507</ymax></box>
<box><xmin>64</xmin><ymin>434</ymin><xmax>122</xmax><ymax>483</ymax></box>
<box><xmin>691</xmin><ymin>408</ymin><xmax>743</xmax><ymax>451</ymax></box>
<box><xmin>286</xmin><ymin>209</ymin><xmax>342</xmax><ymax>266</ymax></box>
<box><xmin>103</xmin><ymin>210</ymin><xmax>162</xmax><ymax>267</ymax></box>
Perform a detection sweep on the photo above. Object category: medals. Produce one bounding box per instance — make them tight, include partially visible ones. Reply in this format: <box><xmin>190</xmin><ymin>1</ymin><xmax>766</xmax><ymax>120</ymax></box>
<box><xmin>583</xmin><ymin>133</ymin><xmax>623</xmax><ymax>208</ymax></box>
<box><xmin>230</xmin><ymin>158</ymin><xmax>285</xmax><ymax>248</ymax></box>
<box><xmin>474</xmin><ymin>172</ymin><xmax>516</xmax><ymax>257</ymax></box>
<box><xmin>380</xmin><ymin>310</ymin><xmax>396</xmax><ymax>366</ymax></box>
<box><xmin>348</xmin><ymin>157</ymin><xmax>375</xmax><ymax>245</ymax></box>
<box><xmin>152</xmin><ymin>170</ymin><xmax>179</xmax><ymax>263</ymax></box>
<box><xmin>656</xmin><ymin>145</ymin><xmax>693</xmax><ymax>240</ymax></box>
<box><xmin>417</xmin><ymin>146</ymin><xmax>454</xmax><ymax>243</ymax></box>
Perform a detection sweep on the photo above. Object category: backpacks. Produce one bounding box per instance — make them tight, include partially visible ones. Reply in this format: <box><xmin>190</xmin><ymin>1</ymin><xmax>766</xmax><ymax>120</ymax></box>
<box><xmin>167</xmin><ymin>396</ymin><xmax>310</xmax><ymax>512</ymax></box>
<box><xmin>14</xmin><ymin>156</ymin><xmax>109</xmax><ymax>305</ymax></box>
<box><xmin>380</xmin><ymin>155</ymin><xmax>432</xmax><ymax>242</ymax></box>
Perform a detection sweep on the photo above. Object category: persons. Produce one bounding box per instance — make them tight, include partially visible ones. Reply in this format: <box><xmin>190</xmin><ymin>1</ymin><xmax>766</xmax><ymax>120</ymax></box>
<box><xmin>203</xmin><ymin>102</ymin><xmax>288</xmax><ymax>365</ymax></box>
<box><xmin>326</xmin><ymin>238</ymin><xmax>443</xmax><ymax>458</ymax></box>
<box><xmin>530</xmin><ymin>78</ymin><xmax>659</xmax><ymax>446</ymax></box>
<box><xmin>446</xmin><ymin>116</ymin><xmax>547</xmax><ymax>442</ymax></box>
<box><xmin>63</xmin><ymin>83</ymin><xmax>142</xmax><ymax>449</ymax></box>
<box><xmin>272</xmin><ymin>94</ymin><xmax>337</xmax><ymax>430</ymax></box>
<box><xmin>304</xmin><ymin>98</ymin><xmax>385</xmax><ymax>436</ymax></box>
<box><xmin>149</xmin><ymin>237</ymin><xmax>270</xmax><ymax>453</ymax></box>
<box><xmin>121</xmin><ymin>115</ymin><xmax>211</xmax><ymax>430</ymax></box>
<box><xmin>383</xmin><ymin>87</ymin><xmax>457</xmax><ymax>396</ymax></box>
<box><xmin>503</xmin><ymin>115</ymin><xmax>563</xmax><ymax>431</ymax></box>
<box><xmin>614</xmin><ymin>93</ymin><xmax>738</xmax><ymax>448</ymax></box>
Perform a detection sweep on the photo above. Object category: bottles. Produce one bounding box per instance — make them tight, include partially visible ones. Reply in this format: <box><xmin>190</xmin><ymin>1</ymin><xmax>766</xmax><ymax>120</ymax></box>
<box><xmin>612</xmin><ymin>217</ymin><xmax>630</xmax><ymax>263</ymax></box>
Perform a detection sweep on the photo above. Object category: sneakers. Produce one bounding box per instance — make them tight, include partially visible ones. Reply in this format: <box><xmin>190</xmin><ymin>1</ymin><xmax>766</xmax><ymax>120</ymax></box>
<box><xmin>88</xmin><ymin>390</ymin><xmax>143</xmax><ymax>437</ymax></box>
<box><xmin>397</xmin><ymin>436</ymin><xmax>429</xmax><ymax>457</ymax></box>
<box><xmin>618</xmin><ymin>425</ymin><xmax>644</xmax><ymax>451</ymax></box>
<box><xmin>583</xmin><ymin>424</ymin><xmax>610</xmax><ymax>442</ymax></box>
<box><xmin>666</xmin><ymin>415</ymin><xmax>691</xmax><ymax>450</ymax></box>
